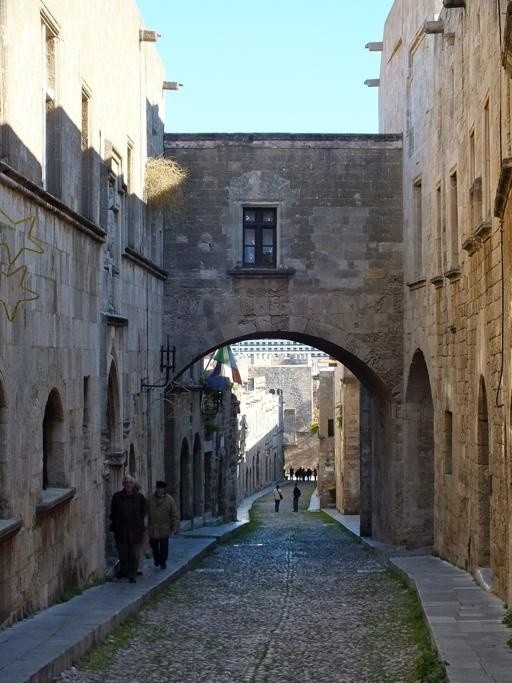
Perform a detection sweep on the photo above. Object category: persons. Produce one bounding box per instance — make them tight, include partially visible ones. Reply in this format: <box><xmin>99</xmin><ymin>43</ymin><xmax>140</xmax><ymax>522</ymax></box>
<box><xmin>107</xmin><ymin>472</ymin><xmax>146</xmax><ymax>585</ymax></box>
<box><xmin>293</xmin><ymin>483</ymin><xmax>300</xmax><ymax>511</ymax></box>
<box><xmin>145</xmin><ymin>480</ymin><xmax>179</xmax><ymax>569</ymax></box>
<box><xmin>283</xmin><ymin>464</ymin><xmax>318</xmax><ymax>481</ymax></box>
<box><xmin>273</xmin><ymin>484</ymin><xmax>282</xmax><ymax>511</ymax></box>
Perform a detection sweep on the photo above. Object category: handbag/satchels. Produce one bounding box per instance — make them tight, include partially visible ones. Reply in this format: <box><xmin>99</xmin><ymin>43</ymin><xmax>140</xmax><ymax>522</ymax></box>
<box><xmin>280</xmin><ymin>495</ymin><xmax>283</xmax><ymax>499</ymax></box>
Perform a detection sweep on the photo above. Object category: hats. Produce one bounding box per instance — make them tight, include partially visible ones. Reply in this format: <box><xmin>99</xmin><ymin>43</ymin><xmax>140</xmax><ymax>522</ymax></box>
<box><xmin>156</xmin><ymin>481</ymin><xmax>166</xmax><ymax>488</ymax></box>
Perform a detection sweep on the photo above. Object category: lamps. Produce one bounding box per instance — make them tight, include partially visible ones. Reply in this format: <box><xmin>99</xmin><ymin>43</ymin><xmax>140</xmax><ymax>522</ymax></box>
<box><xmin>139</xmin><ymin>335</ymin><xmax>176</xmax><ymax>393</ymax></box>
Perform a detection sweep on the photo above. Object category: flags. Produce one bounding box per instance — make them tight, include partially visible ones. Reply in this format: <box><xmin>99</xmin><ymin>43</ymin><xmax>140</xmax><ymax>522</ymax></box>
<box><xmin>207</xmin><ymin>345</ymin><xmax>241</xmax><ymax>390</ymax></box>
